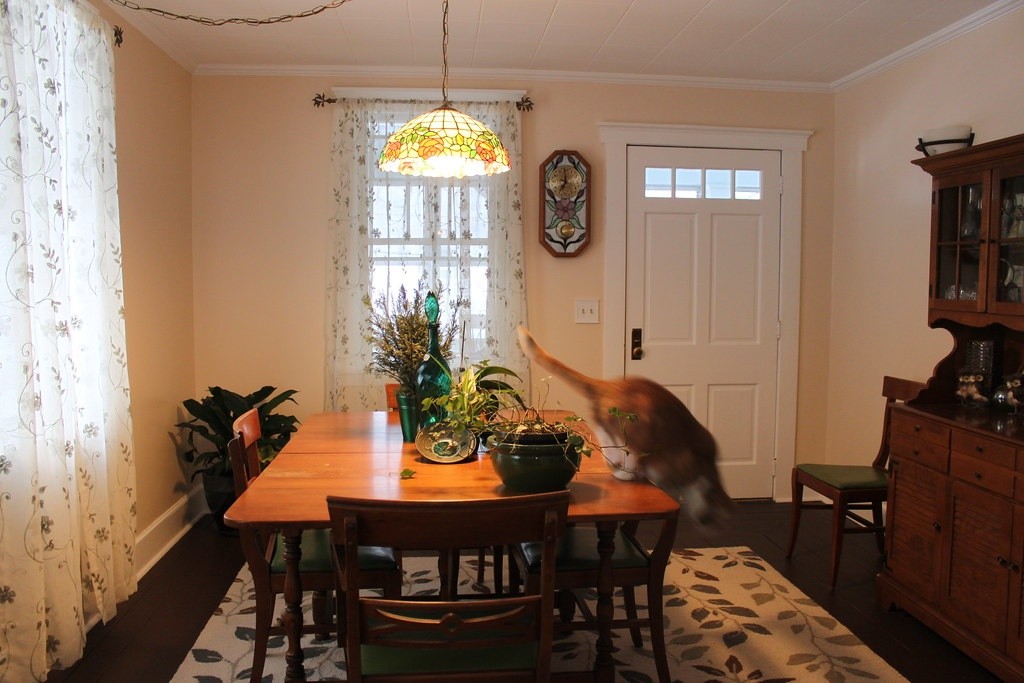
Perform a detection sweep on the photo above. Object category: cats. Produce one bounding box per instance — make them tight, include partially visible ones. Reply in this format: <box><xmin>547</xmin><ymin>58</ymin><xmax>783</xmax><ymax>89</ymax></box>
<box><xmin>516</xmin><ymin>323</ymin><xmax>734</xmax><ymax>535</ymax></box>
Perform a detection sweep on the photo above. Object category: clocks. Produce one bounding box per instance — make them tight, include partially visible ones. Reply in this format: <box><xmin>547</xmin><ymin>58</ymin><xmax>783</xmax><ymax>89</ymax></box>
<box><xmin>539</xmin><ymin>150</ymin><xmax>591</xmax><ymax>258</ymax></box>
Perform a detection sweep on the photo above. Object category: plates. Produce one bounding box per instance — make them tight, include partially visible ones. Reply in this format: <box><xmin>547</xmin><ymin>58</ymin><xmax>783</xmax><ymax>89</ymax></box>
<box><xmin>414</xmin><ymin>422</ymin><xmax>476</xmax><ymax>463</ymax></box>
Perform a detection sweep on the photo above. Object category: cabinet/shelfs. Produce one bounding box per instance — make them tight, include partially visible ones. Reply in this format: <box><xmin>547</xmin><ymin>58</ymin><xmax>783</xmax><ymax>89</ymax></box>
<box><xmin>874</xmin><ymin>133</ymin><xmax>1024</xmax><ymax>683</ymax></box>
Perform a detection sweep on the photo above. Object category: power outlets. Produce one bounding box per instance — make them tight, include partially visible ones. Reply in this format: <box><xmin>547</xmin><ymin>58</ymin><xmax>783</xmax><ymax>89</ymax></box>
<box><xmin>576</xmin><ymin>300</ymin><xmax>599</xmax><ymax>323</ymax></box>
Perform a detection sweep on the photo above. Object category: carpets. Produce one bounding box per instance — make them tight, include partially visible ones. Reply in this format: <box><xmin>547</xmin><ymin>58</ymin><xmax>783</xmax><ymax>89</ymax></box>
<box><xmin>169</xmin><ymin>546</ymin><xmax>912</xmax><ymax>683</ymax></box>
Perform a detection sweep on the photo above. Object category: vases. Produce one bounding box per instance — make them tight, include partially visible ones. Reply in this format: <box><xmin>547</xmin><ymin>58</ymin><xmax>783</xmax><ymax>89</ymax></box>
<box><xmin>916</xmin><ymin>124</ymin><xmax>975</xmax><ymax>157</ymax></box>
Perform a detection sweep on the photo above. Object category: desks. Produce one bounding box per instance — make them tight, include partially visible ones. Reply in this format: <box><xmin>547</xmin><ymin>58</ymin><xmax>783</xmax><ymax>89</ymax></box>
<box><xmin>224</xmin><ymin>411</ymin><xmax>680</xmax><ymax>683</ymax></box>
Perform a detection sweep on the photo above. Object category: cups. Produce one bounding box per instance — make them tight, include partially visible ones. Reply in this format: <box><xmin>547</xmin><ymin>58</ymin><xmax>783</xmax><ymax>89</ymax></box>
<box><xmin>945</xmin><ymin>284</ymin><xmax>976</xmax><ymax>301</ymax></box>
<box><xmin>970</xmin><ymin>340</ymin><xmax>993</xmax><ymax>396</ymax></box>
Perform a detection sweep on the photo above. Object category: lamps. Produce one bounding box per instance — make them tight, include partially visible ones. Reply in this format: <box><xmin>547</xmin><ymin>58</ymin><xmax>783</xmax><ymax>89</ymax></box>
<box><xmin>379</xmin><ymin>0</ymin><xmax>511</xmax><ymax>177</ymax></box>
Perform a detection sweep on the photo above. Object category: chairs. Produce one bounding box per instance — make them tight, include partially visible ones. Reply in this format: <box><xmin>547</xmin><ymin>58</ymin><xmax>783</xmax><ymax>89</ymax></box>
<box><xmin>784</xmin><ymin>376</ymin><xmax>926</xmax><ymax>590</ymax></box>
<box><xmin>227</xmin><ymin>384</ymin><xmax>680</xmax><ymax>683</ymax></box>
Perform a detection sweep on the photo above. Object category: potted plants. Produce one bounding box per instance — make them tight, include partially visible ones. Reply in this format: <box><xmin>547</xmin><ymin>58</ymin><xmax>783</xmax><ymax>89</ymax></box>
<box><xmin>175</xmin><ymin>386</ymin><xmax>299</xmax><ymax>535</ymax></box>
<box><xmin>363</xmin><ymin>277</ymin><xmax>639</xmax><ymax>493</ymax></box>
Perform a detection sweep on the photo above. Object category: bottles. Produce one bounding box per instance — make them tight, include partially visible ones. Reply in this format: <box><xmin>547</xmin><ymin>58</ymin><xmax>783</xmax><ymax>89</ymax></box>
<box><xmin>957</xmin><ymin>187</ymin><xmax>981</xmax><ymax>240</ymax></box>
<box><xmin>1001</xmin><ymin>186</ymin><xmax>1024</xmax><ymax>238</ymax></box>
<box><xmin>414</xmin><ymin>291</ymin><xmax>452</xmax><ymax>431</ymax></box>
